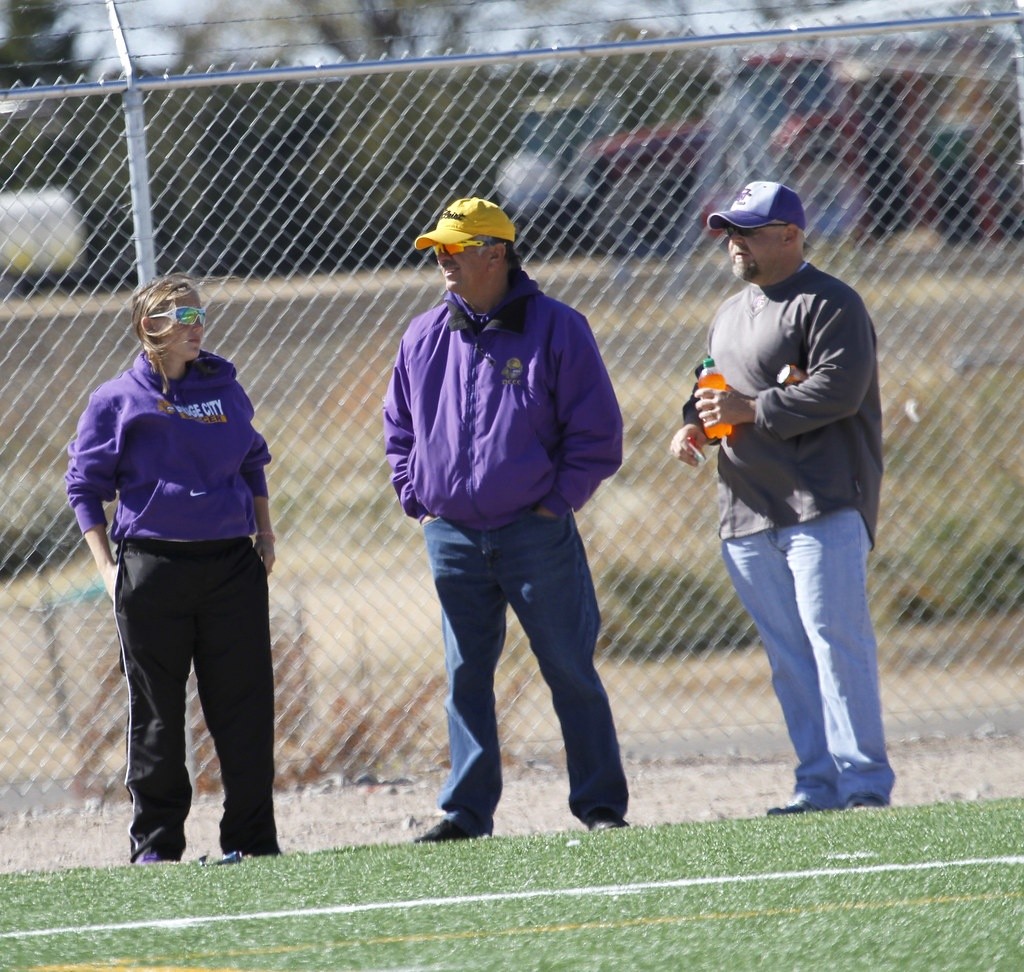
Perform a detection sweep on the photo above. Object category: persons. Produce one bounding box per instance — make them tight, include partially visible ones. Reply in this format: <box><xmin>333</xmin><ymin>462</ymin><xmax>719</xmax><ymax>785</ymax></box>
<box><xmin>65</xmin><ymin>275</ymin><xmax>280</xmax><ymax>863</ymax></box>
<box><xmin>382</xmin><ymin>197</ymin><xmax>622</xmax><ymax>840</ymax></box>
<box><xmin>671</xmin><ymin>181</ymin><xmax>893</xmax><ymax>815</ymax></box>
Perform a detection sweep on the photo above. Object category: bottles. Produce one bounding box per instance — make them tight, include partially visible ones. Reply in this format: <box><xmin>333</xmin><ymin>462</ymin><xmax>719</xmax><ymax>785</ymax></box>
<box><xmin>698</xmin><ymin>358</ymin><xmax>732</xmax><ymax>438</ymax></box>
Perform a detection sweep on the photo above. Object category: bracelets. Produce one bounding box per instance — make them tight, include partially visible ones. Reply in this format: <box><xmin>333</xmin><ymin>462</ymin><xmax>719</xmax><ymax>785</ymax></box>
<box><xmin>256</xmin><ymin>530</ymin><xmax>275</xmax><ymax>542</ymax></box>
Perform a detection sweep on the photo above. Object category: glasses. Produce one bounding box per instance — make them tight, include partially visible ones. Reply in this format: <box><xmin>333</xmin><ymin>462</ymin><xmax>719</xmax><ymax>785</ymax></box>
<box><xmin>148</xmin><ymin>306</ymin><xmax>206</xmax><ymax>327</ymax></box>
<box><xmin>433</xmin><ymin>239</ymin><xmax>509</xmax><ymax>256</ymax></box>
<box><xmin>724</xmin><ymin>223</ymin><xmax>789</xmax><ymax>238</ymax></box>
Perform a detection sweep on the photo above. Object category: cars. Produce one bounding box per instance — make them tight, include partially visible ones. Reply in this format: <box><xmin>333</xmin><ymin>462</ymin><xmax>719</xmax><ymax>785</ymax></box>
<box><xmin>570</xmin><ymin>45</ymin><xmax>1023</xmax><ymax>244</ymax></box>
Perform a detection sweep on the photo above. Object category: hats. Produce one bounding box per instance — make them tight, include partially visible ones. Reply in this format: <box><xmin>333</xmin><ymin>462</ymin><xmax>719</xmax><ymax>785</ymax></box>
<box><xmin>706</xmin><ymin>181</ymin><xmax>807</xmax><ymax>232</ymax></box>
<box><xmin>415</xmin><ymin>196</ymin><xmax>516</xmax><ymax>251</ymax></box>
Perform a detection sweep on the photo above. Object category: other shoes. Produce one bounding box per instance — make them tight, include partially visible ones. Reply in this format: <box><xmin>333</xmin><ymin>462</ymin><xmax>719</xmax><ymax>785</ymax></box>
<box><xmin>586</xmin><ymin>806</ymin><xmax>631</xmax><ymax>833</ymax></box>
<box><xmin>844</xmin><ymin>793</ymin><xmax>882</xmax><ymax>807</ymax></box>
<box><xmin>766</xmin><ymin>793</ymin><xmax>820</xmax><ymax>814</ymax></box>
<box><xmin>136</xmin><ymin>851</ymin><xmax>169</xmax><ymax>862</ymax></box>
<box><xmin>415</xmin><ymin>820</ymin><xmax>470</xmax><ymax>844</ymax></box>
